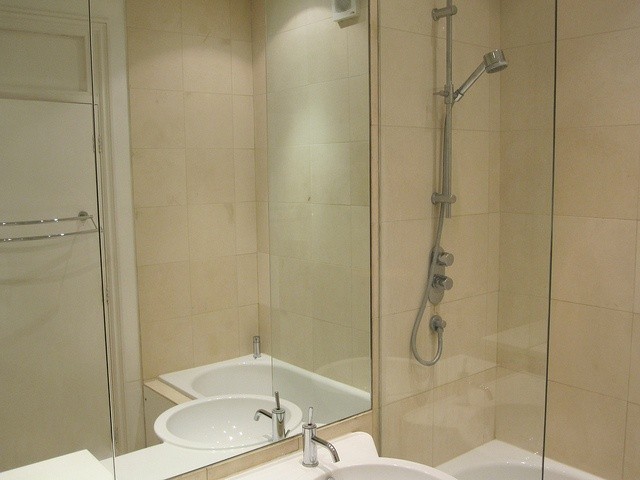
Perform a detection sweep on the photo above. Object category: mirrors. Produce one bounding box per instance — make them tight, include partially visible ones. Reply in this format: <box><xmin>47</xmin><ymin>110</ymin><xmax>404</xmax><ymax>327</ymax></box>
<box><xmin>1</xmin><ymin>1</ymin><xmax>373</xmax><ymax>480</ymax></box>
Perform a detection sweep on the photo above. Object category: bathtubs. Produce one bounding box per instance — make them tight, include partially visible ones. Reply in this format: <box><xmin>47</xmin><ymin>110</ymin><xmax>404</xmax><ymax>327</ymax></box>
<box><xmin>157</xmin><ymin>353</ymin><xmax>371</xmax><ymax>424</ymax></box>
<box><xmin>453</xmin><ymin>461</ymin><xmax>588</xmax><ymax>480</ymax></box>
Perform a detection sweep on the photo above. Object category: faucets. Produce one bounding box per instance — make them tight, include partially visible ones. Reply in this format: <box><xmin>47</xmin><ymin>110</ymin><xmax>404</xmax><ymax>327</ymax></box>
<box><xmin>300</xmin><ymin>406</ymin><xmax>341</xmax><ymax>468</ymax></box>
<box><xmin>253</xmin><ymin>390</ymin><xmax>288</xmax><ymax>439</ymax></box>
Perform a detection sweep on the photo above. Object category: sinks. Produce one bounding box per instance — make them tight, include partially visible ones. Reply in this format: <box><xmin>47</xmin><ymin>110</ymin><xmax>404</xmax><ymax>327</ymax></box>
<box><xmin>153</xmin><ymin>396</ymin><xmax>292</xmax><ymax>445</ymax></box>
<box><xmin>311</xmin><ymin>463</ymin><xmax>443</xmax><ymax>480</ymax></box>
